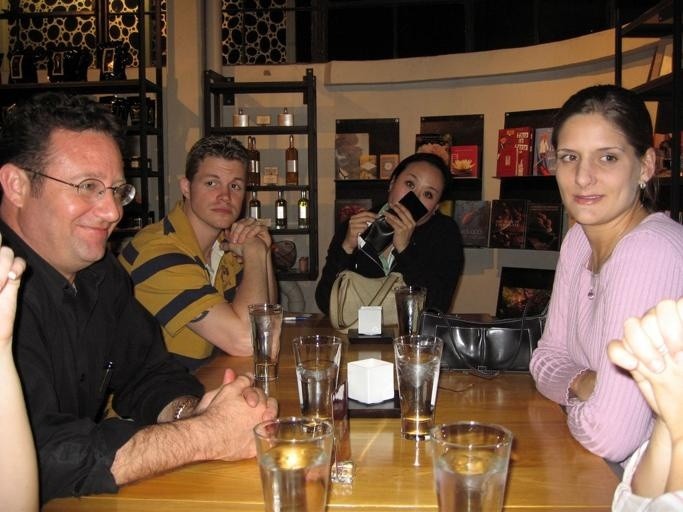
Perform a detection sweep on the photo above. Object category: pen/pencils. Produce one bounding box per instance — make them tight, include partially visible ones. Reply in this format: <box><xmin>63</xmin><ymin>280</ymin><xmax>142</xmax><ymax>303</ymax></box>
<box><xmin>98</xmin><ymin>361</ymin><xmax>113</xmax><ymax>392</ymax></box>
<box><xmin>282</xmin><ymin>317</ymin><xmax>308</xmax><ymax>320</ymax></box>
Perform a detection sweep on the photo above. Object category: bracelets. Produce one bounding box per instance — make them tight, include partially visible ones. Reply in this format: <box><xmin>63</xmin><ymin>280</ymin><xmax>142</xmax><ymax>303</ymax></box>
<box><xmin>0</xmin><ymin>92</ymin><xmax>280</xmax><ymax>503</ymax></box>
<box><xmin>171</xmin><ymin>397</ymin><xmax>199</xmax><ymax>421</ymax></box>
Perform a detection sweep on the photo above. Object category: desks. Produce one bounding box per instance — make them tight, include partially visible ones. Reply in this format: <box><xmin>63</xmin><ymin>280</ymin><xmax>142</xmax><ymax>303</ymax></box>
<box><xmin>40</xmin><ymin>327</ymin><xmax>622</xmax><ymax>512</ymax></box>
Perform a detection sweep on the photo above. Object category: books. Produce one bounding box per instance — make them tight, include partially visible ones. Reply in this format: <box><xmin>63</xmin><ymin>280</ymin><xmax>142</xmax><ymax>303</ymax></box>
<box><xmin>416</xmin><ymin>127</ymin><xmax>567</xmax><ymax>251</ymax></box>
<box><xmin>649</xmin><ymin>132</ymin><xmax>672</xmax><ymax>178</ymax></box>
<box><xmin>334</xmin><ymin>133</ymin><xmax>398</xmax><ymax>225</ymax></box>
<box><xmin>448</xmin><ymin>269</ymin><xmax>555</xmax><ymax>317</ymax></box>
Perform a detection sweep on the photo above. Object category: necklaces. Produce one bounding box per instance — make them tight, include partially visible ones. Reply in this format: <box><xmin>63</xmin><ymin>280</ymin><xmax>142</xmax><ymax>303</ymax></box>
<box><xmin>585</xmin><ymin>203</ymin><xmax>642</xmax><ymax>300</ymax></box>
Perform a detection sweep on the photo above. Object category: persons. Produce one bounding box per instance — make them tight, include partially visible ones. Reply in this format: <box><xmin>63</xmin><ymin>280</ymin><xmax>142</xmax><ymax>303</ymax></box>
<box><xmin>527</xmin><ymin>83</ymin><xmax>682</xmax><ymax>481</ymax></box>
<box><xmin>0</xmin><ymin>230</ymin><xmax>41</xmax><ymax>512</ymax></box>
<box><xmin>604</xmin><ymin>295</ymin><xmax>682</xmax><ymax>512</ymax></box>
<box><xmin>117</xmin><ymin>133</ymin><xmax>277</xmax><ymax>369</ymax></box>
<box><xmin>314</xmin><ymin>152</ymin><xmax>464</xmax><ymax>327</ymax></box>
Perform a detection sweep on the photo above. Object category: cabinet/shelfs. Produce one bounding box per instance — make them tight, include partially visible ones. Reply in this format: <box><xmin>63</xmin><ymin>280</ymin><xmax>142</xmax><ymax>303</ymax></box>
<box><xmin>615</xmin><ymin>1</ymin><xmax>682</xmax><ymax>224</ymax></box>
<box><xmin>203</xmin><ymin>68</ymin><xmax>318</xmax><ymax>280</ymax></box>
<box><xmin>1</xmin><ymin>0</ymin><xmax>161</xmax><ymax>243</ymax></box>
<box><xmin>330</xmin><ymin>174</ymin><xmax>563</xmax><ymax>252</ymax></box>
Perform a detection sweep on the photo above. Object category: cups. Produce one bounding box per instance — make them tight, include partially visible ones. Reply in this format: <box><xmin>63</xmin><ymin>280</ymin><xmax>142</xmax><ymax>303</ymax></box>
<box><xmin>289</xmin><ymin>335</ymin><xmax>343</xmax><ymax>419</ymax></box>
<box><xmin>251</xmin><ymin>418</ymin><xmax>329</xmax><ymax>512</ymax></box>
<box><xmin>391</xmin><ymin>333</ymin><xmax>443</xmax><ymax>441</ymax></box>
<box><xmin>428</xmin><ymin>419</ymin><xmax>513</xmax><ymax>512</ymax></box>
<box><xmin>246</xmin><ymin>301</ymin><xmax>281</xmax><ymax>382</ymax></box>
<box><xmin>393</xmin><ymin>285</ymin><xmax>428</xmax><ymax>336</ymax></box>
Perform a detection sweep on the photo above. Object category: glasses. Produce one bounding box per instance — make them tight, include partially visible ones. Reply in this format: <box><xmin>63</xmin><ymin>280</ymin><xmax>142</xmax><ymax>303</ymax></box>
<box><xmin>19</xmin><ymin>167</ymin><xmax>137</xmax><ymax>207</ymax></box>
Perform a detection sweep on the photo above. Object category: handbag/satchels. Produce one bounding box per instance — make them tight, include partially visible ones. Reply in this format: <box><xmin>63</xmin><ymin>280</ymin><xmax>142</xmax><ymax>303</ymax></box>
<box><xmin>417</xmin><ymin>311</ymin><xmax>547</xmax><ymax>373</ymax></box>
<box><xmin>330</xmin><ymin>270</ymin><xmax>407</xmax><ymax>335</ymax></box>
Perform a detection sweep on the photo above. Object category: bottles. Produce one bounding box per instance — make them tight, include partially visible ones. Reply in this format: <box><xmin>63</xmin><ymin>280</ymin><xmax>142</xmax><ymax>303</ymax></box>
<box><xmin>243</xmin><ymin>136</ymin><xmax>301</xmax><ymax>186</ymax></box>
<box><xmin>244</xmin><ymin>189</ymin><xmax>309</xmax><ymax>229</ymax></box>
